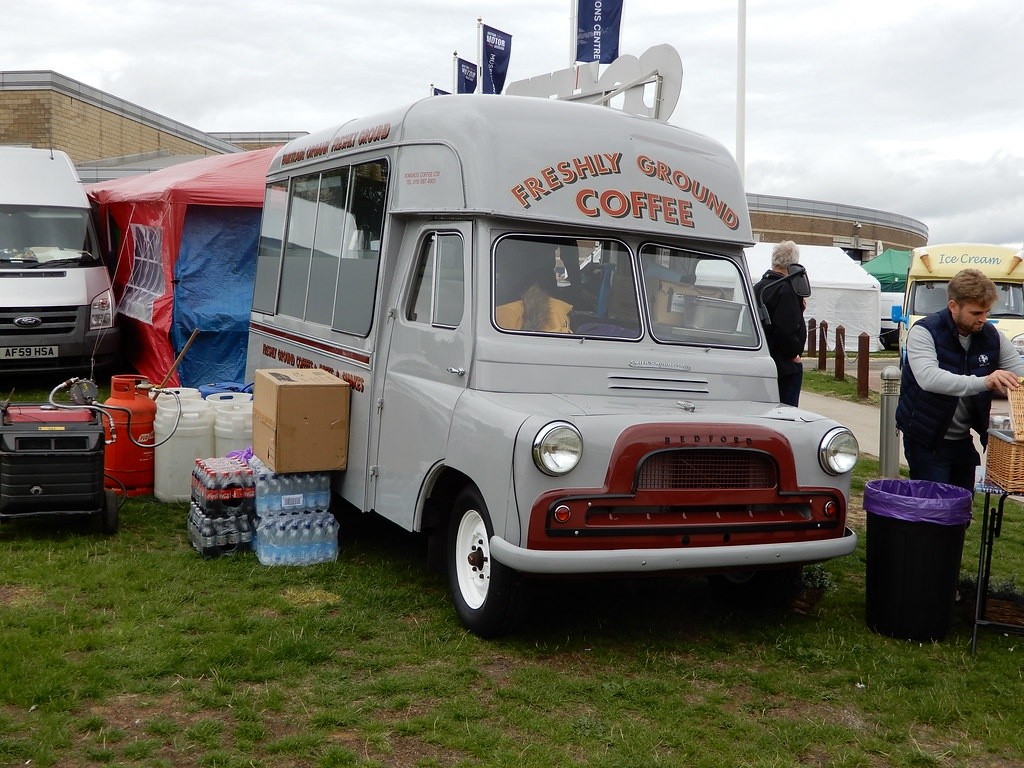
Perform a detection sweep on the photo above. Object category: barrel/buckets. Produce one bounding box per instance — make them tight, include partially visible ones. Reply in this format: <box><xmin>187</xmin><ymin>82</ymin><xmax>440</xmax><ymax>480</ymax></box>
<box><xmin>148</xmin><ymin>381</ymin><xmax>254</xmax><ymax>503</ymax></box>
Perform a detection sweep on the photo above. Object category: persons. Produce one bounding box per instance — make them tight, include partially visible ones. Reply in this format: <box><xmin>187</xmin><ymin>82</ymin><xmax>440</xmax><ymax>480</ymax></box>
<box><xmin>752</xmin><ymin>241</ymin><xmax>808</xmax><ymax>407</ymax></box>
<box><xmin>894</xmin><ymin>268</ymin><xmax>1024</xmax><ymax>491</ymax></box>
<box><xmin>497</xmin><ymin>236</ymin><xmax>583</xmax><ymax>303</ymax></box>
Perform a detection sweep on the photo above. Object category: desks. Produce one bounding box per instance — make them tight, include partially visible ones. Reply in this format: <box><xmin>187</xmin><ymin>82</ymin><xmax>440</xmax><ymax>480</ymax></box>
<box><xmin>654</xmin><ymin>325</ymin><xmax>753</xmax><ymax>343</ymax></box>
<box><xmin>971</xmin><ymin>465</ymin><xmax>1024</xmax><ymax>661</ymax></box>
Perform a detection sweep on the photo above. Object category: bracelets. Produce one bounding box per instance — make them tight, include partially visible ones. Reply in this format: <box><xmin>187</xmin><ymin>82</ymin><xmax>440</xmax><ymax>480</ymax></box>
<box><xmin>794</xmin><ymin>354</ymin><xmax>799</xmax><ymax>361</ymax></box>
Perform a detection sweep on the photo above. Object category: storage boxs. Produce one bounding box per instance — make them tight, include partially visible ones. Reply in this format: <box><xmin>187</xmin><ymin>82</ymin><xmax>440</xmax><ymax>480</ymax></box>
<box><xmin>253</xmin><ymin>369</ymin><xmax>351</xmax><ymax>474</ymax></box>
<box><xmin>679</xmin><ymin>293</ymin><xmax>748</xmax><ymax>334</ymax></box>
<box><xmin>607</xmin><ymin>275</ymin><xmax>722</xmax><ymax>326</ymax></box>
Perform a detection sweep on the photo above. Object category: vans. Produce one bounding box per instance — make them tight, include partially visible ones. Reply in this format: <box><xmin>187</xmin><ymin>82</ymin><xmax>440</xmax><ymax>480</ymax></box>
<box><xmin>242</xmin><ymin>43</ymin><xmax>860</xmax><ymax>639</ymax></box>
<box><xmin>891</xmin><ymin>243</ymin><xmax>1024</xmax><ymax>373</ymax></box>
<box><xmin>0</xmin><ymin>146</ymin><xmax>116</xmax><ymax>380</ymax></box>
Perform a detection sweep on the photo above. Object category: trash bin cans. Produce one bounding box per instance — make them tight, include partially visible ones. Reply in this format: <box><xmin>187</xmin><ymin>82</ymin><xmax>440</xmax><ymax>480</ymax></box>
<box><xmin>863</xmin><ymin>479</ymin><xmax>973</xmax><ymax>644</ymax></box>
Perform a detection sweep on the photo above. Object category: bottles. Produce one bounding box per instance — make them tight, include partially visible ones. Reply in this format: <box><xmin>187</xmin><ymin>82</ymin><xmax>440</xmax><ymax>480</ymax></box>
<box><xmin>187</xmin><ymin>453</ymin><xmax>338</xmax><ymax>567</ymax></box>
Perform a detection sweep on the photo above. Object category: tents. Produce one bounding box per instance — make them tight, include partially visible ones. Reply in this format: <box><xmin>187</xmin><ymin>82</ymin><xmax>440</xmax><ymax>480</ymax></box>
<box><xmin>86</xmin><ymin>141</ymin><xmax>286</xmax><ymax>376</ymax></box>
<box><xmin>860</xmin><ymin>248</ymin><xmax>912</xmax><ymax>327</ymax></box>
<box><xmin>684</xmin><ymin>245</ymin><xmax>885</xmax><ymax>353</ymax></box>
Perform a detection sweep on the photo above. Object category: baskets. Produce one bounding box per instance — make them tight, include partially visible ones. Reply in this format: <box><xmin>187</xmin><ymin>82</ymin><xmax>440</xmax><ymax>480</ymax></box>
<box><xmin>985</xmin><ymin>376</ymin><xmax>1024</xmax><ymax>492</ymax></box>
